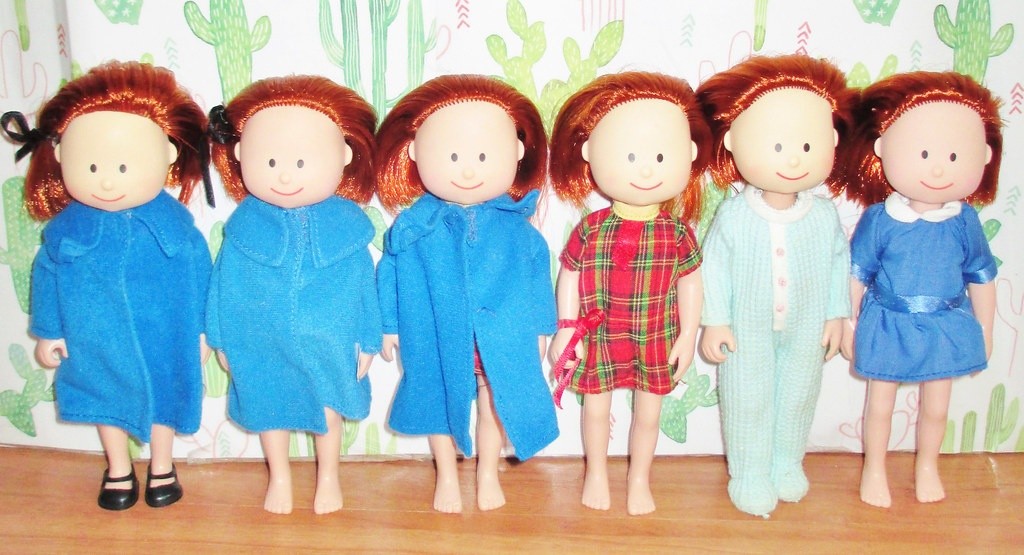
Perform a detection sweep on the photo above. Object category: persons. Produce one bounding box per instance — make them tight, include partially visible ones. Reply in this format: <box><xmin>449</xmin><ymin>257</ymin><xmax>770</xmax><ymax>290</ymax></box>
<box><xmin>839</xmin><ymin>70</ymin><xmax>1005</xmax><ymax>509</ymax></box>
<box><xmin>540</xmin><ymin>70</ymin><xmax>710</xmax><ymax>517</ymax></box>
<box><xmin>0</xmin><ymin>57</ymin><xmax>214</xmax><ymax>514</ymax></box>
<box><xmin>366</xmin><ymin>72</ymin><xmax>560</xmax><ymax>514</ymax></box>
<box><xmin>693</xmin><ymin>51</ymin><xmax>858</xmax><ymax>523</ymax></box>
<box><xmin>197</xmin><ymin>71</ymin><xmax>384</xmax><ymax>517</ymax></box>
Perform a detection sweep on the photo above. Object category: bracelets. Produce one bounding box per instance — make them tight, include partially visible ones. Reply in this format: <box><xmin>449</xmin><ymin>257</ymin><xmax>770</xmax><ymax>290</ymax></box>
<box><xmin>553</xmin><ymin>308</ymin><xmax>606</xmax><ymax>411</ymax></box>
